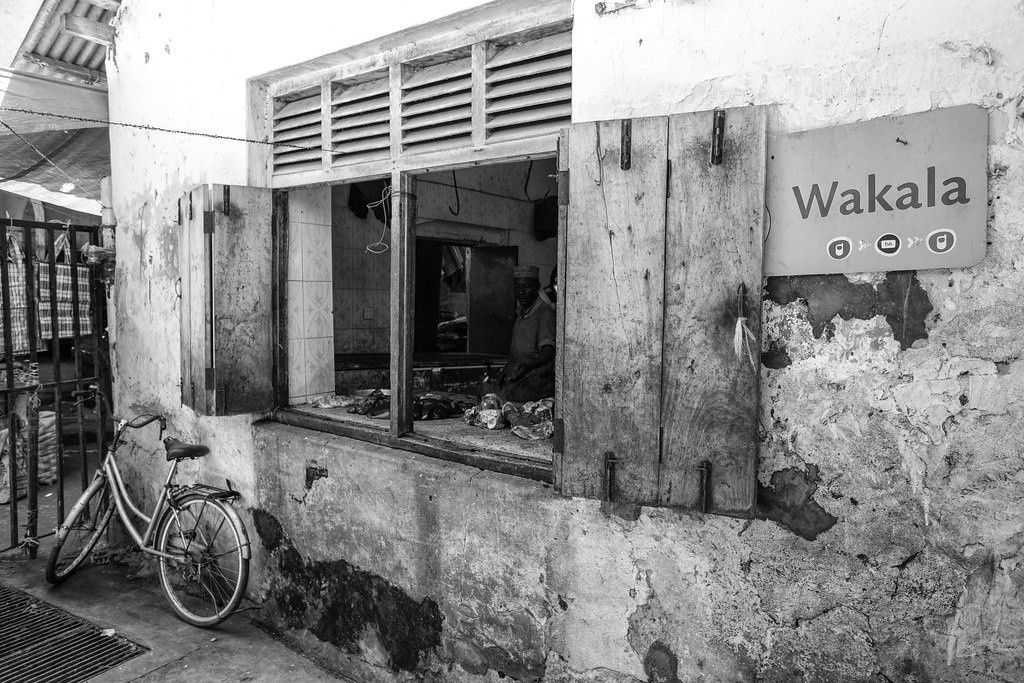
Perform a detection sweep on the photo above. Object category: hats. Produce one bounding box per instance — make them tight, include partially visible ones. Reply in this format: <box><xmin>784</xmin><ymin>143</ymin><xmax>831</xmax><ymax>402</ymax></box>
<box><xmin>513</xmin><ymin>265</ymin><xmax>540</xmax><ymax>281</ymax></box>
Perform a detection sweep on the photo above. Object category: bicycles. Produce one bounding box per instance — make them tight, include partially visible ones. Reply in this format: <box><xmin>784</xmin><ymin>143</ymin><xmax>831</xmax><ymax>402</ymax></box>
<box><xmin>45</xmin><ymin>385</ymin><xmax>252</xmax><ymax>628</ymax></box>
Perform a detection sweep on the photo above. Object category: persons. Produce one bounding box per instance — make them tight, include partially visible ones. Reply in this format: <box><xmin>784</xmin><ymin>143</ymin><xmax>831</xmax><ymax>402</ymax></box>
<box><xmin>499</xmin><ymin>265</ymin><xmax>556</xmax><ymax>405</ymax></box>
